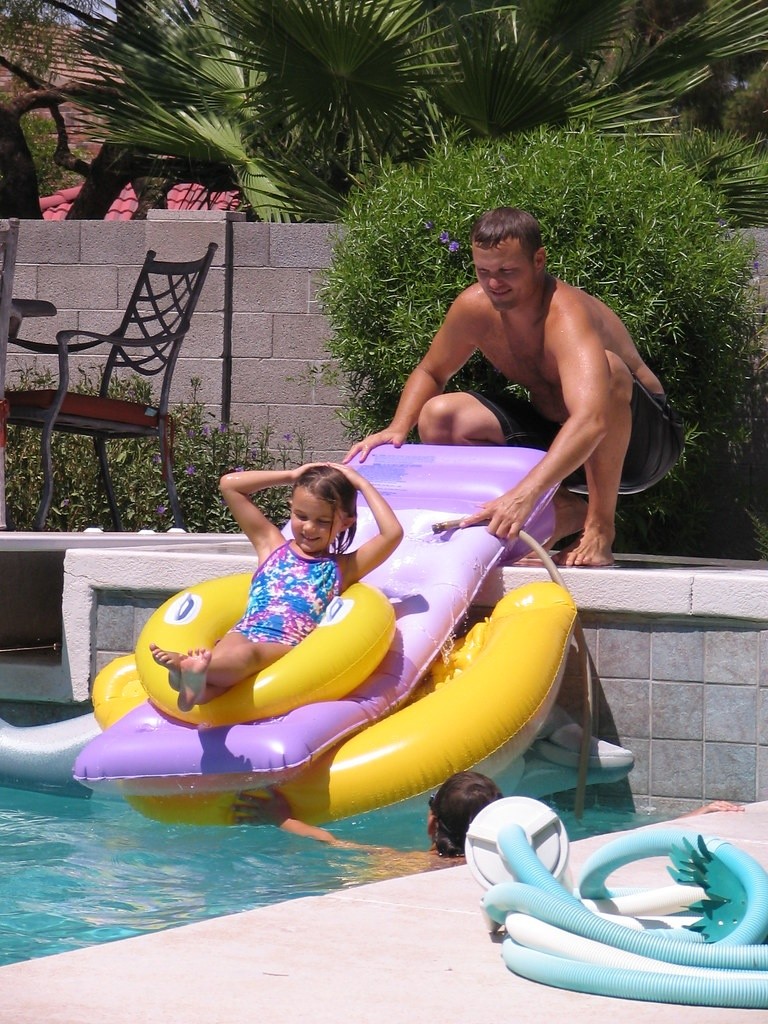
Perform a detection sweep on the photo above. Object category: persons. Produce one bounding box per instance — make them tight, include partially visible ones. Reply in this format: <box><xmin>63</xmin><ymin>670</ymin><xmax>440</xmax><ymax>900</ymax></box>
<box><xmin>231</xmin><ymin>770</ymin><xmax>747</xmax><ymax>859</ymax></box>
<box><xmin>343</xmin><ymin>207</ymin><xmax>684</xmax><ymax>566</ymax></box>
<box><xmin>150</xmin><ymin>461</ymin><xmax>405</xmax><ymax>712</ymax></box>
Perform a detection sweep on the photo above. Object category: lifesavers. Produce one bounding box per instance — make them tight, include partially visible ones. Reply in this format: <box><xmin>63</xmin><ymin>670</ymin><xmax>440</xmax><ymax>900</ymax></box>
<box><xmin>133</xmin><ymin>571</ymin><xmax>399</xmax><ymax>730</ymax></box>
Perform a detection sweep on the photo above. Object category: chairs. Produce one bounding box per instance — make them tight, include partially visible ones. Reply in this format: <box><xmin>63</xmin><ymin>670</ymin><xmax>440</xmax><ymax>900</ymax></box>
<box><xmin>1</xmin><ymin>241</ymin><xmax>217</xmax><ymax>531</ymax></box>
